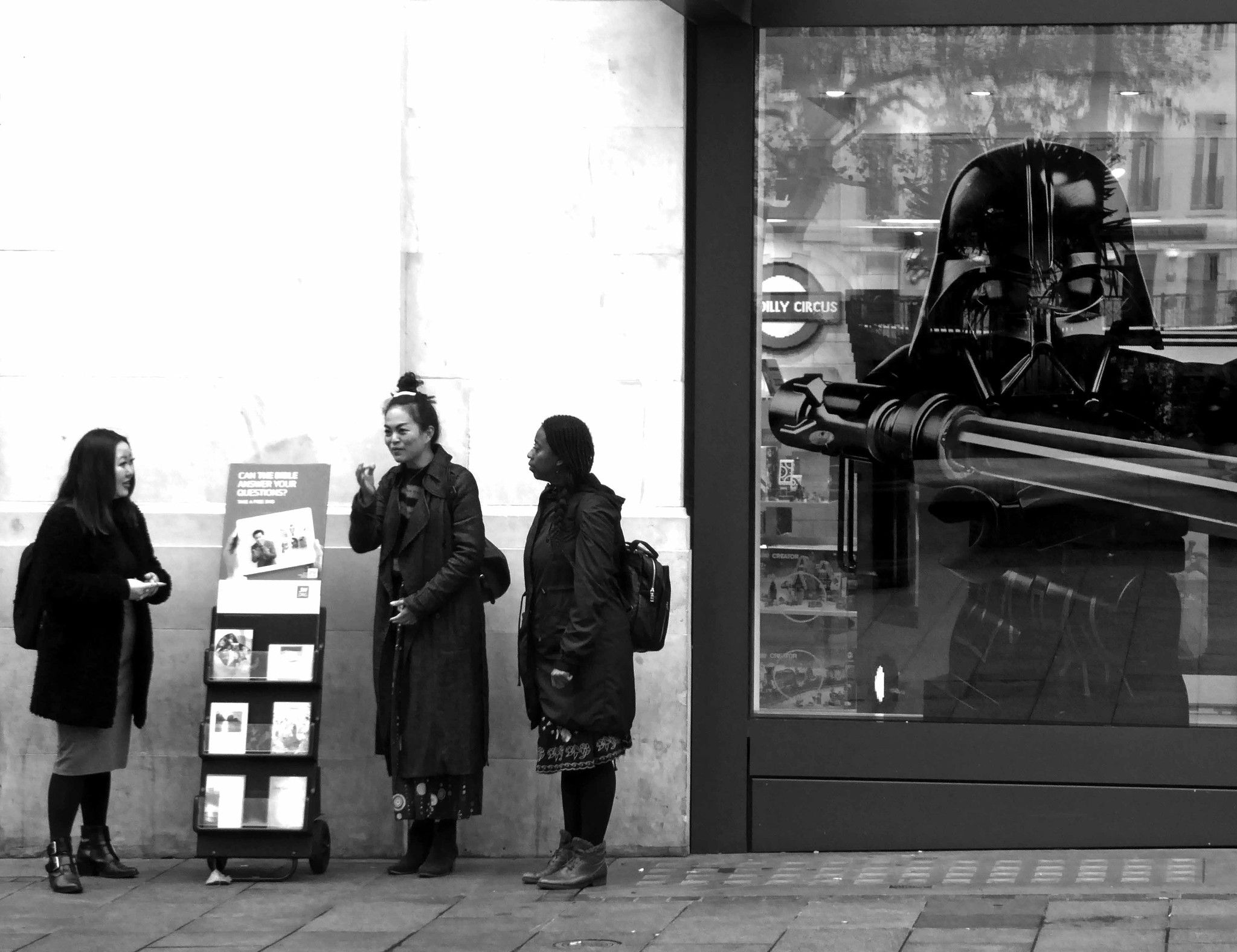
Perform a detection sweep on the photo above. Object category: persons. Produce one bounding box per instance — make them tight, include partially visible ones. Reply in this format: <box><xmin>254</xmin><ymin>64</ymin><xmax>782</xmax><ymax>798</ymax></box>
<box><xmin>515</xmin><ymin>413</ymin><xmax>635</xmax><ymax>890</ymax></box>
<box><xmin>251</xmin><ymin>530</ymin><xmax>278</xmax><ymax>567</ymax></box>
<box><xmin>347</xmin><ymin>370</ymin><xmax>494</xmax><ymax>878</ymax></box>
<box><xmin>26</xmin><ymin>426</ymin><xmax>173</xmax><ymax>894</ymax></box>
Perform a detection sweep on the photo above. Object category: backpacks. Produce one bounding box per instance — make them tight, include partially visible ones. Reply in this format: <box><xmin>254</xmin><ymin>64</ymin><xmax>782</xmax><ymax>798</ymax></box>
<box><xmin>575</xmin><ymin>492</ymin><xmax>671</xmax><ymax>653</ymax></box>
<box><xmin>444</xmin><ymin>459</ymin><xmax>511</xmax><ymax>605</ymax></box>
<box><xmin>13</xmin><ymin>542</ymin><xmax>48</xmax><ymax>650</ymax></box>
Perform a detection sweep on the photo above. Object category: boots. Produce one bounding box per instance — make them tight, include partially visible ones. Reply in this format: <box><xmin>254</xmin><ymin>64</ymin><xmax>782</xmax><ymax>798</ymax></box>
<box><xmin>75</xmin><ymin>825</ymin><xmax>140</xmax><ymax>878</ymax></box>
<box><xmin>417</xmin><ymin>824</ymin><xmax>458</xmax><ymax>877</ymax></box>
<box><xmin>521</xmin><ymin>829</ymin><xmax>574</xmax><ymax>884</ymax></box>
<box><xmin>536</xmin><ymin>838</ymin><xmax>608</xmax><ymax>890</ymax></box>
<box><xmin>45</xmin><ymin>835</ymin><xmax>83</xmax><ymax>893</ymax></box>
<box><xmin>387</xmin><ymin>822</ymin><xmax>432</xmax><ymax>876</ymax></box>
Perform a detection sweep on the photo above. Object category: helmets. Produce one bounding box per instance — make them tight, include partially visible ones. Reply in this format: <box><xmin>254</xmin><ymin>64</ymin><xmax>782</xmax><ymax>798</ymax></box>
<box><xmin>905</xmin><ymin>138</ymin><xmax>1167</xmax><ymax>360</ymax></box>
